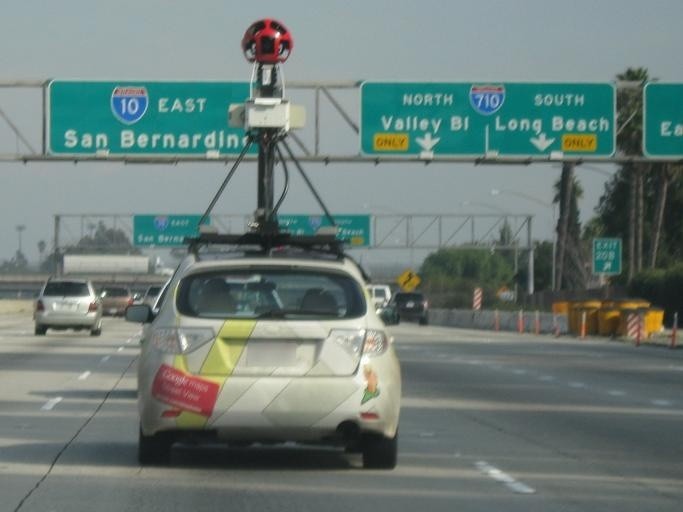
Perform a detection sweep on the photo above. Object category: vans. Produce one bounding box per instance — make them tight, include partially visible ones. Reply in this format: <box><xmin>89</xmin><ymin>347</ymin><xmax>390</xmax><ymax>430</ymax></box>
<box><xmin>365</xmin><ymin>284</ymin><xmax>390</xmax><ymax>315</ymax></box>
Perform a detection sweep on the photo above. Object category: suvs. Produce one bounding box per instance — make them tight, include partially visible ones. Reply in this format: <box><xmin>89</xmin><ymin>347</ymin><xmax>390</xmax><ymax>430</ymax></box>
<box><xmin>385</xmin><ymin>291</ymin><xmax>428</xmax><ymax>325</ymax></box>
<box><xmin>33</xmin><ymin>277</ymin><xmax>165</xmax><ymax>336</ymax></box>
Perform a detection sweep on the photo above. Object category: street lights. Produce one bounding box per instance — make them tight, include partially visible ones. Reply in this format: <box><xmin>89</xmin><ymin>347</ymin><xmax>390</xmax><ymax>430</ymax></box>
<box><xmin>489</xmin><ymin>189</ymin><xmax>557</xmax><ymax>292</ymax></box>
<box><xmin>16</xmin><ymin>225</ymin><xmax>26</xmax><ymax>250</ymax></box>
<box><xmin>456</xmin><ymin>199</ymin><xmax>521</xmax><ymax>305</ymax></box>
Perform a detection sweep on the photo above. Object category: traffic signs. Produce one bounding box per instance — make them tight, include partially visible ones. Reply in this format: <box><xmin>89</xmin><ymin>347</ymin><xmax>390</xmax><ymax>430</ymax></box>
<box><xmin>641</xmin><ymin>82</ymin><xmax>682</xmax><ymax>161</ymax></box>
<box><xmin>272</xmin><ymin>212</ymin><xmax>367</xmax><ymax>250</ymax></box>
<box><xmin>592</xmin><ymin>236</ymin><xmax>621</xmax><ymax>276</ymax></box>
<box><xmin>133</xmin><ymin>215</ymin><xmax>214</xmax><ymax>245</ymax></box>
<box><xmin>46</xmin><ymin>80</ymin><xmax>259</xmax><ymax>160</ymax></box>
<box><xmin>360</xmin><ymin>81</ymin><xmax>615</xmax><ymax>164</ymax></box>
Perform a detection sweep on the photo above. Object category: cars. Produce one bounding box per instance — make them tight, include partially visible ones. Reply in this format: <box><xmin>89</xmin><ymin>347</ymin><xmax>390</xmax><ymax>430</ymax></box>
<box><xmin>132</xmin><ymin>249</ymin><xmax>402</xmax><ymax>474</ymax></box>
